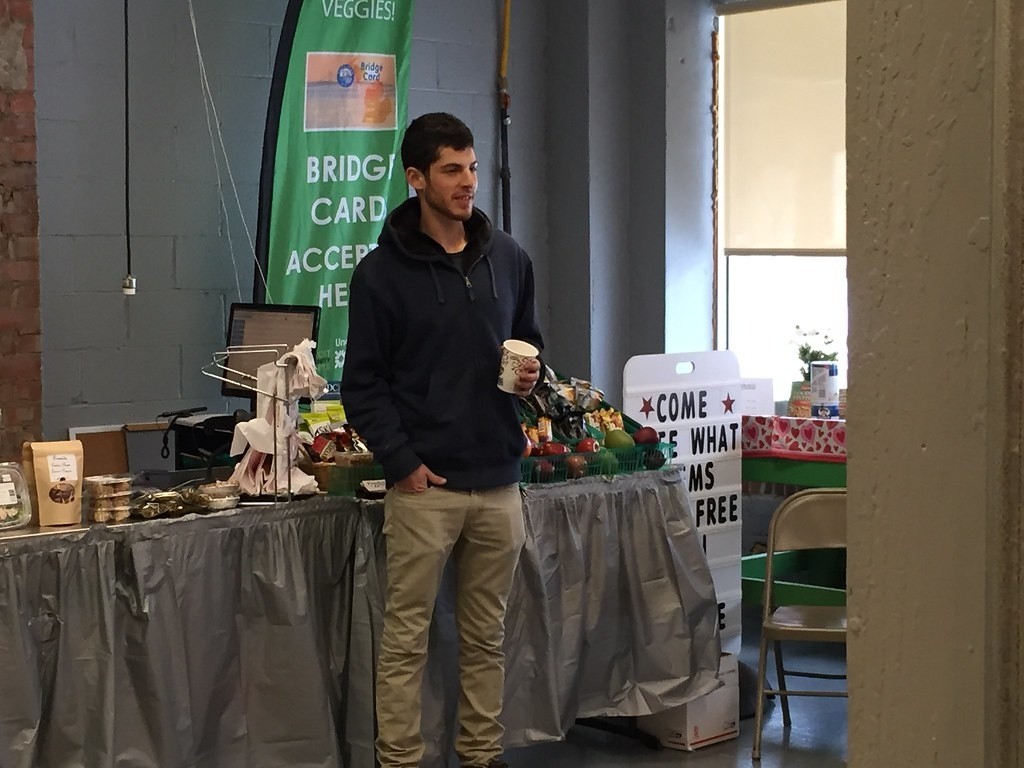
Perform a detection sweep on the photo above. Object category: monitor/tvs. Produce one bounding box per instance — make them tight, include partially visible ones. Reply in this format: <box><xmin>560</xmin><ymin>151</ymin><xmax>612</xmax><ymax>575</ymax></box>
<box><xmin>222</xmin><ymin>302</ymin><xmax>322</xmax><ymax>412</ymax></box>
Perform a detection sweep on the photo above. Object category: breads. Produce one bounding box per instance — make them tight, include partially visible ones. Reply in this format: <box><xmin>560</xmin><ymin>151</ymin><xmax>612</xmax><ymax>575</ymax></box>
<box><xmin>88</xmin><ymin>481</ymin><xmax>131</xmax><ymax>523</ymax></box>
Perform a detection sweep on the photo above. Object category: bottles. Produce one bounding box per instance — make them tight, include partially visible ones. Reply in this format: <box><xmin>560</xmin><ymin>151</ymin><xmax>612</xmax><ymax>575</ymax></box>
<box><xmin>810</xmin><ymin>360</ymin><xmax>839</xmax><ymax>420</ymax></box>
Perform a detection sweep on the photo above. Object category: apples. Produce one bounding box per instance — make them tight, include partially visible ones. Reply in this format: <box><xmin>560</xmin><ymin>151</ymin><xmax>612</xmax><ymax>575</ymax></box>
<box><xmin>522</xmin><ymin>426</ymin><xmax>661</xmax><ymax>482</ymax></box>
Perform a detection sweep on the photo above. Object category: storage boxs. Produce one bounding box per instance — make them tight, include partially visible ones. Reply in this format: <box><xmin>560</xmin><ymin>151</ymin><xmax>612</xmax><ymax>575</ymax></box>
<box><xmin>636</xmin><ymin>651</ymin><xmax>740</xmax><ymax>751</ymax></box>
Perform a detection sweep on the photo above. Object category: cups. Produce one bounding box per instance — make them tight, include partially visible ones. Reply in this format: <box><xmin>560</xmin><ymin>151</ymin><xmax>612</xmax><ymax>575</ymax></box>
<box><xmin>497</xmin><ymin>339</ymin><xmax>539</xmax><ymax>394</ymax></box>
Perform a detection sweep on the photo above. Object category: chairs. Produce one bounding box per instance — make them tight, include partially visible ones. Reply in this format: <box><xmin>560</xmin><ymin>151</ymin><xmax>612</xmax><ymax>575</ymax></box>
<box><xmin>753</xmin><ymin>486</ymin><xmax>849</xmax><ymax>760</ymax></box>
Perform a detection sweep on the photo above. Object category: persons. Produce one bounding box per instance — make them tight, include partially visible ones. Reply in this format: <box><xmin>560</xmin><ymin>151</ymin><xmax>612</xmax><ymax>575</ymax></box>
<box><xmin>341</xmin><ymin>112</ymin><xmax>546</xmax><ymax>768</ymax></box>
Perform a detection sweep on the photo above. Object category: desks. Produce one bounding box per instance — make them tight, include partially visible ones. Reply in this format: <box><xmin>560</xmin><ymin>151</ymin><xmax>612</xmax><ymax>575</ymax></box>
<box><xmin>0</xmin><ymin>468</ymin><xmax>724</xmax><ymax>768</ymax></box>
<box><xmin>743</xmin><ymin>415</ymin><xmax>847</xmax><ymax>485</ymax></box>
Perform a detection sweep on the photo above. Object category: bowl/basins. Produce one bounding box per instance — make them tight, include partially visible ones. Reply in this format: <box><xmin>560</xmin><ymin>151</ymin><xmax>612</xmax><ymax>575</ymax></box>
<box><xmin>0</xmin><ymin>465</ymin><xmax>31</xmax><ymax>531</ymax></box>
<box><xmin>210</xmin><ymin>497</ymin><xmax>241</xmax><ymax>509</ymax></box>
<box><xmin>201</xmin><ymin>484</ymin><xmax>238</xmax><ymax>496</ymax></box>
<box><xmin>84</xmin><ymin>472</ymin><xmax>135</xmax><ymax>524</ymax></box>
